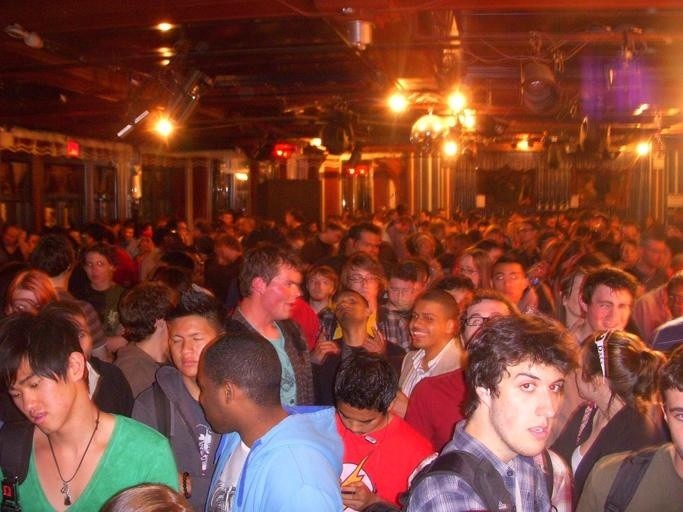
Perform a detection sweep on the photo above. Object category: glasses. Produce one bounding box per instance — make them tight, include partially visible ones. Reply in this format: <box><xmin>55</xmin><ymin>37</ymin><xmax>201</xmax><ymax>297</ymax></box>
<box><xmin>348</xmin><ymin>275</ymin><xmax>374</xmax><ymax>284</ymax></box>
<box><xmin>463</xmin><ymin>317</ymin><xmax>490</xmax><ymax>328</ymax></box>
<box><xmin>594</xmin><ymin>329</ymin><xmax>611</xmax><ymax>384</ymax></box>
<box><xmin>459</xmin><ymin>269</ymin><xmax>477</xmax><ymax>274</ymax></box>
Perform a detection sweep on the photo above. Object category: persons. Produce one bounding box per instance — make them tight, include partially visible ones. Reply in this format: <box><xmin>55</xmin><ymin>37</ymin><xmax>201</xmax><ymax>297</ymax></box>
<box><xmin>1</xmin><ymin>190</ymin><xmax>683</xmax><ymax>511</ymax></box>
